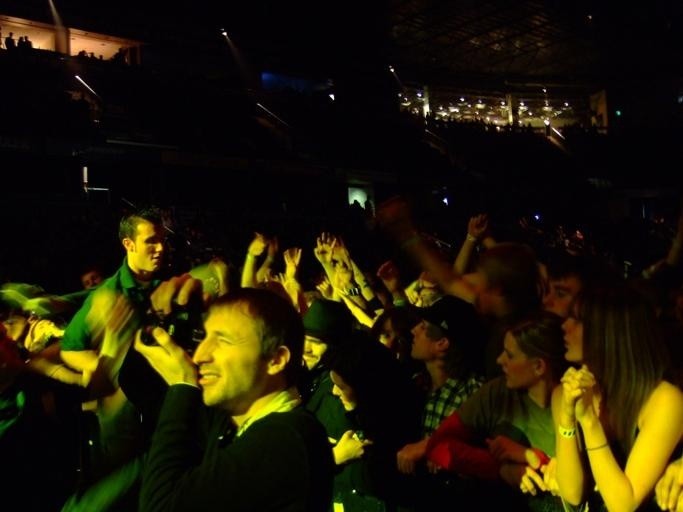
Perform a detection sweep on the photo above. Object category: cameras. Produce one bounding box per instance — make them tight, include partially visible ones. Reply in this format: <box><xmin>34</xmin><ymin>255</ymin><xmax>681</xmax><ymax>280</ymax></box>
<box><xmin>127</xmin><ymin>281</ymin><xmax>204</xmax><ymax>347</ymax></box>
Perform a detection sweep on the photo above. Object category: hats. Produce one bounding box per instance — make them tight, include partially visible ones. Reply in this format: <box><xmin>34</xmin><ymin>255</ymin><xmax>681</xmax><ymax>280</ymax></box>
<box><xmin>405</xmin><ymin>295</ymin><xmax>477</xmax><ymax>340</ymax></box>
